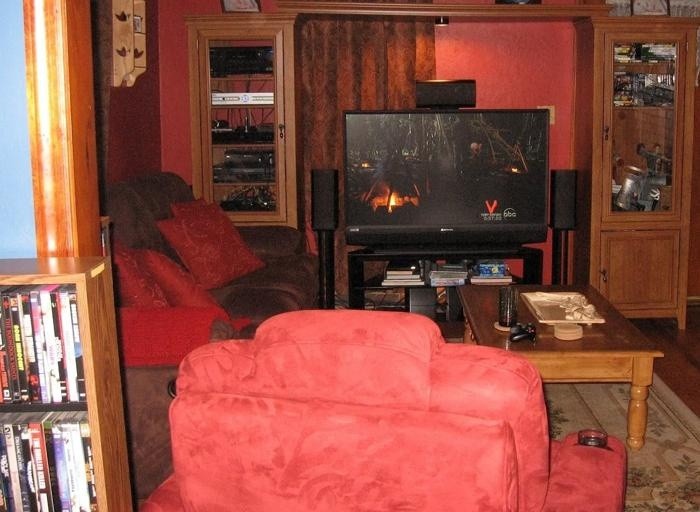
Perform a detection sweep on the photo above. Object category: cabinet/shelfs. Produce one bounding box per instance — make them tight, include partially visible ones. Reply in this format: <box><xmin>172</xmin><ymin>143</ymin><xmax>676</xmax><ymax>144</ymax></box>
<box><xmin>573</xmin><ymin>22</ymin><xmax>697</xmax><ymax>331</ymax></box>
<box><xmin>0</xmin><ymin>256</ymin><xmax>131</xmax><ymax>512</ymax></box>
<box><xmin>345</xmin><ymin>245</ymin><xmax>545</xmax><ymax>340</ymax></box>
<box><xmin>110</xmin><ymin>0</ymin><xmax>149</xmax><ymax>91</ymax></box>
<box><xmin>187</xmin><ymin>23</ymin><xmax>299</xmax><ymax>228</ymax></box>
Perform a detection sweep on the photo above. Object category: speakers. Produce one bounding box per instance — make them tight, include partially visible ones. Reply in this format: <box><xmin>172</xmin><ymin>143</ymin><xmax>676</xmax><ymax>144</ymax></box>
<box><xmin>549</xmin><ymin>169</ymin><xmax>579</xmax><ymax>229</ymax></box>
<box><xmin>415</xmin><ymin>79</ymin><xmax>475</xmax><ymax>108</ymax></box>
<box><xmin>310</xmin><ymin>167</ymin><xmax>338</xmax><ymax>230</ymax></box>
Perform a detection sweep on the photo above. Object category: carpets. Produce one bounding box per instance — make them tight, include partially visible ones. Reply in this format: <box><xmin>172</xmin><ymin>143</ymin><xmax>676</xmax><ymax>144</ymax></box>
<box><xmin>542</xmin><ymin>373</ymin><xmax>699</xmax><ymax>511</ymax></box>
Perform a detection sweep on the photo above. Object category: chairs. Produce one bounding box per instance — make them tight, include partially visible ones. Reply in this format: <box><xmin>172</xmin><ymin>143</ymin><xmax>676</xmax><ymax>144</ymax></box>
<box><xmin>139</xmin><ymin>308</ymin><xmax>632</xmax><ymax>511</ymax></box>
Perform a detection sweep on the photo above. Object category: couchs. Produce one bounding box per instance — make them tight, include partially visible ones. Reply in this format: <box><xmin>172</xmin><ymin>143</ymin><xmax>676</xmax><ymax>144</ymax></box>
<box><xmin>102</xmin><ymin>170</ymin><xmax>321</xmax><ymax>399</ymax></box>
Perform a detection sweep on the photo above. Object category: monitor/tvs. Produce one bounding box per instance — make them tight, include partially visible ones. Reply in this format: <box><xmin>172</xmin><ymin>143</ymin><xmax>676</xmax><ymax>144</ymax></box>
<box><xmin>342</xmin><ymin>108</ymin><xmax>550</xmax><ymax>252</ymax></box>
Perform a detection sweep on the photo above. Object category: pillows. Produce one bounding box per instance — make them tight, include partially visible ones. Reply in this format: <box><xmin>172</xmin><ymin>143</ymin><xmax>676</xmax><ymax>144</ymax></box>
<box><xmin>110</xmin><ymin>193</ymin><xmax>268</xmax><ymax>321</ymax></box>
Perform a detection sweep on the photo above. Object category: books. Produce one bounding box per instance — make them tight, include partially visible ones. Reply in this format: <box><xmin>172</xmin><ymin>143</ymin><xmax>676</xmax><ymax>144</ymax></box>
<box><xmin>0</xmin><ymin>283</ymin><xmax>102</xmax><ymax>512</ymax></box>
<box><xmin>379</xmin><ymin>255</ymin><xmax>515</xmax><ymax>286</ymax></box>
<box><xmin>613</xmin><ymin>42</ymin><xmax>676</xmax><ymax>107</ymax></box>
